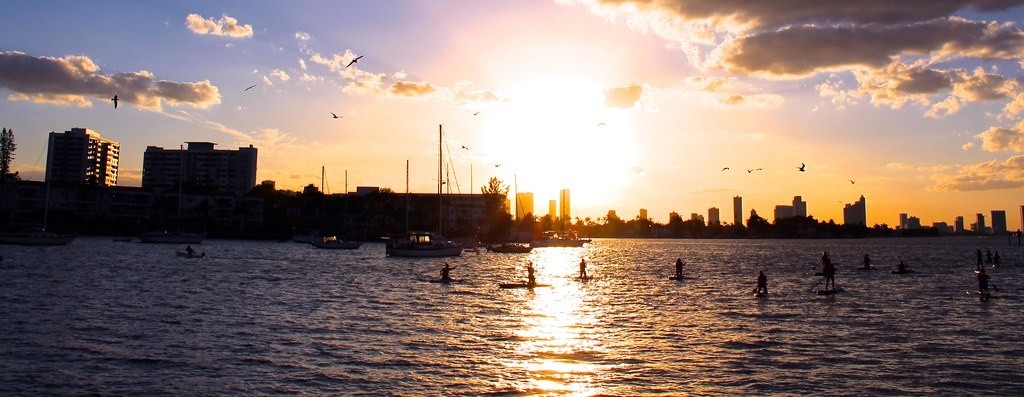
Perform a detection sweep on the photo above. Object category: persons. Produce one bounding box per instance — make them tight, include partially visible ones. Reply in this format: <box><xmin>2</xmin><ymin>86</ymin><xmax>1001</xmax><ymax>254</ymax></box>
<box><xmin>978</xmin><ymin>269</ymin><xmax>990</xmax><ymax>292</ymax></box>
<box><xmin>898</xmin><ymin>261</ymin><xmax>905</xmax><ymax>271</ymax></box>
<box><xmin>528</xmin><ymin>262</ymin><xmax>535</xmax><ymax>283</ymax></box>
<box><xmin>439</xmin><ymin>264</ymin><xmax>457</xmax><ymax>279</ymax></box>
<box><xmin>820</xmin><ymin>250</ymin><xmax>828</xmax><ymax>266</ymax></box>
<box><xmin>986</xmin><ymin>250</ymin><xmax>993</xmax><ymax>261</ymax></box>
<box><xmin>186</xmin><ymin>245</ymin><xmax>195</xmax><ymax>255</ymax></box>
<box><xmin>580</xmin><ymin>259</ymin><xmax>587</xmax><ymax>277</ymax></box>
<box><xmin>863</xmin><ymin>254</ymin><xmax>869</xmax><ymax>268</ymax></box>
<box><xmin>676</xmin><ymin>259</ymin><xmax>686</xmax><ymax>277</ymax></box>
<box><xmin>758</xmin><ymin>271</ymin><xmax>767</xmax><ymax>293</ymax></box>
<box><xmin>822</xmin><ymin>258</ymin><xmax>836</xmax><ymax>290</ymax></box>
<box><xmin>976</xmin><ymin>249</ymin><xmax>983</xmax><ymax>269</ymax></box>
<box><xmin>993</xmin><ymin>251</ymin><xmax>1000</xmax><ymax>264</ymax></box>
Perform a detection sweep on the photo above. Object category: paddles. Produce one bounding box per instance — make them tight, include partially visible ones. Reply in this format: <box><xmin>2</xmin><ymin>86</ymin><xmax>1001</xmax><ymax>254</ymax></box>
<box><xmin>433</xmin><ymin>272</ymin><xmax>444</xmax><ymax>282</ymax></box>
<box><xmin>674</xmin><ymin>261</ymin><xmax>686</xmax><ymax>276</ymax></box>
<box><xmin>812</xmin><ymin>252</ymin><xmax>830</xmax><ymax>270</ymax></box>
<box><xmin>808</xmin><ymin>268</ymin><xmax>838</xmax><ymax>291</ymax></box>
<box><xmin>753</xmin><ymin>269</ymin><xmax>768</xmax><ymax>293</ymax></box>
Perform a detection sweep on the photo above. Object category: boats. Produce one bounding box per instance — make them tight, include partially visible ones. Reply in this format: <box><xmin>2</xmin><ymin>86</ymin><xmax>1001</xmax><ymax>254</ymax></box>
<box><xmin>111</xmin><ymin>238</ymin><xmax>132</xmax><ymax>242</ymax></box>
<box><xmin>498</xmin><ymin>280</ymin><xmax>552</xmax><ymax>289</ymax></box>
<box><xmin>744</xmin><ymin>265</ymin><xmax>915</xmax><ymax>301</ymax></box>
<box><xmin>668</xmin><ymin>274</ymin><xmax>699</xmax><ymax>281</ymax></box>
<box><xmin>572</xmin><ymin>276</ymin><xmax>596</xmax><ymax>282</ymax></box>
<box><xmin>428</xmin><ymin>277</ymin><xmax>462</xmax><ymax>284</ymax></box>
<box><xmin>177</xmin><ymin>248</ymin><xmax>208</xmax><ymax>259</ymax></box>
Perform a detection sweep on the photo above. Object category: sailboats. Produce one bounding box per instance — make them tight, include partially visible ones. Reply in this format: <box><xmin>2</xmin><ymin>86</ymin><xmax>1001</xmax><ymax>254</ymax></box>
<box><xmin>135</xmin><ymin>145</ymin><xmax>208</xmax><ymax>244</ymax></box>
<box><xmin>385</xmin><ymin>124</ymin><xmax>594</xmax><ymax>258</ymax></box>
<box><xmin>291</xmin><ymin>166</ymin><xmax>363</xmax><ymax>251</ymax></box>
<box><xmin>0</xmin><ymin>129</ymin><xmax>78</xmax><ymax>245</ymax></box>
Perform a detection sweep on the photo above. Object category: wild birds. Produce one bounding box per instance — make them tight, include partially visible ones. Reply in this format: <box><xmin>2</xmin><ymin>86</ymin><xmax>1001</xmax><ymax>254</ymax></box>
<box><xmin>346</xmin><ymin>55</ymin><xmax>363</xmax><ymax>67</ymax></box>
<box><xmin>473</xmin><ymin>111</ymin><xmax>479</xmax><ymax>115</ymax></box>
<box><xmin>111</xmin><ymin>94</ymin><xmax>118</xmax><ymax>109</ymax></box>
<box><xmin>244</xmin><ymin>84</ymin><xmax>256</xmax><ymax>91</ymax></box>
<box><xmin>748</xmin><ymin>169</ymin><xmax>753</xmax><ymax>173</ymax></box>
<box><xmin>721</xmin><ymin>167</ymin><xmax>729</xmax><ymax>171</ymax></box>
<box><xmin>599</xmin><ymin>123</ymin><xmax>605</xmax><ymax>125</ymax></box>
<box><xmin>755</xmin><ymin>168</ymin><xmax>762</xmax><ymax>171</ymax></box>
<box><xmin>849</xmin><ymin>180</ymin><xmax>855</xmax><ymax>184</ymax></box>
<box><xmin>796</xmin><ymin>162</ymin><xmax>806</xmax><ymax>172</ymax></box>
<box><xmin>461</xmin><ymin>145</ymin><xmax>469</xmax><ymax>151</ymax></box>
<box><xmin>330</xmin><ymin>112</ymin><xmax>338</xmax><ymax>118</ymax></box>
